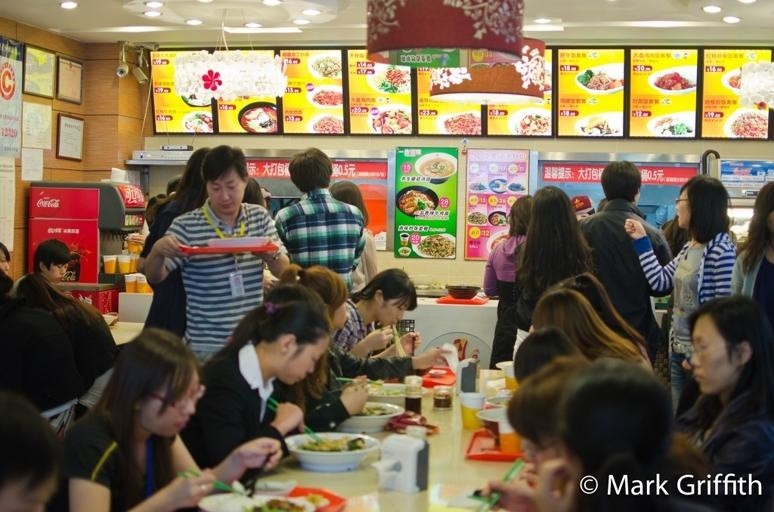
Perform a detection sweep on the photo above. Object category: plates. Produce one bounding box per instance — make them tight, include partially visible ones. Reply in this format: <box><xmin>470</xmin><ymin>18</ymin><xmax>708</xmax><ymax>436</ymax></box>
<box><xmin>725</xmin><ymin>106</ymin><xmax>769</xmax><ymax>138</ymax></box>
<box><xmin>575</xmin><ymin>111</ymin><xmax>623</xmax><ymax>137</ymax></box>
<box><xmin>468</xmin><ymin>182</ymin><xmax>488</xmax><ymax>193</ymax></box>
<box><xmin>366</xmin><ymin>63</ymin><xmax>411</xmax><ymax>95</ymax></box>
<box><xmin>575</xmin><ymin>62</ymin><xmax>624</xmax><ymax>95</ymax></box>
<box><xmin>367</xmin><ymin>104</ymin><xmax>412</xmax><ymax>134</ymax></box>
<box><xmin>486</xmin><ymin>229</ymin><xmax>510</xmax><ymax>253</ymax></box>
<box><xmin>207</xmin><ymin>235</ymin><xmax>273</xmax><ymax>248</ymax></box>
<box><xmin>508</xmin><ymin>108</ymin><xmax>552</xmax><ymax>135</ymax></box>
<box><xmin>720</xmin><ymin>68</ymin><xmax>742</xmax><ymax>93</ymax></box>
<box><xmin>307</xmin><ymin>112</ymin><xmax>344</xmax><ymax>134</ymax></box>
<box><xmin>647</xmin><ymin>66</ymin><xmax>697</xmax><ymax>95</ymax></box>
<box><xmin>307</xmin><ymin>52</ymin><xmax>342</xmax><ymax>80</ymax></box>
<box><xmin>436</xmin><ymin>111</ymin><xmax>481</xmax><ymax>135</ymax></box>
<box><xmin>181</xmin><ymin>110</ymin><xmax>214</xmax><ymax>133</ymax></box>
<box><xmin>467</xmin><ymin>210</ymin><xmax>488</xmax><ymax>226</ymax></box>
<box><xmin>505</xmin><ymin>181</ymin><xmax>527</xmax><ymax>194</ymax></box>
<box><xmin>647</xmin><ymin>111</ymin><xmax>695</xmax><ymax>137</ymax></box>
<box><xmin>307</xmin><ymin>84</ymin><xmax>343</xmax><ymax>109</ymax></box>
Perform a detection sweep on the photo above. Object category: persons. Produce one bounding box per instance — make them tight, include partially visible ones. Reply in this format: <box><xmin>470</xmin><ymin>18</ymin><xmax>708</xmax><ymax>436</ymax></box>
<box><xmin>482</xmin><ymin>195</ymin><xmax>533</xmax><ymax>370</ymax></box>
<box><xmin>659</xmin><ymin>213</ymin><xmax>738</xmax><ymax>329</ymax></box>
<box><xmin>12</xmin><ymin>239</ymin><xmax>72</xmax><ymax>303</ymax></box>
<box><xmin>242</xmin><ymin>177</ymin><xmax>272</xmax><ymax>210</ymax></box>
<box><xmin>145</xmin><ymin>194</ymin><xmax>167</xmax><ymax>228</ymax></box>
<box><xmin>16</xmin><ymin>273</ymin><xmax>117</xmax><ymax>383</ymax></box>
<box><xmin>513</xmin><ymin>186</ymin><xmax>594</xmax><ymax>330</ymax></box>
<box><xmin>624</xmin><ymin>173</ymin><xmax>737</xmax><ymax>416</ymax></box>
<box><xmin>574</xmin><ymin>160</ymin><xmax>672</xmax><ymax>346</ymax></box>
<box><xmin>275</xmin><ymin>147</ymin><xmax>366</xmax><ymax>295</ymax></box>
<box><xmin>331</xmin><ymin>268</ymin><xmax>421</xmax><ymax>363</ymax></box>
<box><xmin>731</xmin><ymin>181</ymin><xmax>774</xmax><ymax>315</ymax></box>
<box><xmin>544</xmin><ymin>272</ymin><xmax>651</xmax><ymax>362</ymax></box>
<box><xmin>156</xmin><ymin>175</ymin><xmax>182</xmax><ymax>214</ymax></box>
<box><xmin>135</xmin><ymin>147</ymin><xmax>210</xmax><ymax>340</ymax></box>
<box><xmin>685</xmin><ymin>294</ymin><xmax>774</xmax><ymax>512</ymax></box>
<box><xmin>60</xmin><ymin>327</ymin><xmax>282</xmax><ymax>512</ymax></box>
<box><xmin>0</xmin><ymin>242</ymin><xmax>10</xmax><ymax>275</ymax></box>
<box><xmin>328</xmin><ymin>180</ymin><xmax>378</xmax><ymax>295</ymax></box>
<box><xmin>265</xmin><ymin>284</ymin><xmax>329</xmax><ymax>413</ymax></box>
<box><xmin>530</xmin><ymin>288</ymin><xmax>652</xmax><ymax>370</ymax></box>
<box><xmin>279</xmin><ymin>262</ymin><xmax>452</xmax><ymax>430</ymax></box>
<box><xmin>0</xmin><ymin>268</ymin><xmax>86</xmax><ymax>412</ymax></box>
<box><xmin>143</xmin><ymin>144</ymin><xmax>290</xmax><ymax>367</ymax></box>
<box><xmin>476</xmin><ymin>358</ymin><xmax>590</xmax><ymax>512</ymax></box>
<box><xmin>512</xmin><ymin>327</ymin><xmax>582</xmax><ymax>382</ymax></box>
<box><xmin>182</xmin><ymin>300</ymin><xmax>333</xmax><ymax>484</ymax></box>
<box><xmin>0</xmin><ymin>392</ymin><xmax>62</xmax><ymax>512</ymax></box>
<box><xmin>533</xmin><ymin>357</ymin><xmax>705</xmax><ymax>511</ymax></box>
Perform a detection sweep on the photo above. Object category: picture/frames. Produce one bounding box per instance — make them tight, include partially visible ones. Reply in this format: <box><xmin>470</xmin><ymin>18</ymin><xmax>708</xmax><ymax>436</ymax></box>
<box><xmin>56</xmin><ymin>54</ymin><xmax>84</xmax><ymax>105</ymax></box>
<box><xmin>56</xmin><ymin>111</ymin><xmax>84</xmax><ymax>163</ymax></box>
<box><xmin>22</xmin><ymin>43</ymin><xmax>57</xmax><ymax>99</ymax></box>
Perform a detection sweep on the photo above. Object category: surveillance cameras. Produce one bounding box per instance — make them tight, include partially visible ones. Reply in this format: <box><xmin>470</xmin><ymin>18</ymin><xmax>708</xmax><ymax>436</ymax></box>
<box><xmin>131</xmin><ymin>67</ymin><xmax>149</xmax><ymax>85</ymax></box>
<box><xmin>116</xmin><ymin>64</ymin><xmax>128</xmax><ymax>78</ymax></box>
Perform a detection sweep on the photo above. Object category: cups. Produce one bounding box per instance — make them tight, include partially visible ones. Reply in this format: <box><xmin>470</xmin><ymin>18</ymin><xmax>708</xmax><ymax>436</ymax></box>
<box><xmin>102</xmin><ymin>255</ymin><xmax>117</xmax><ymax>273</ymax></box>
<box><xmin>137</xmin><ymin>275</ymin><xmax>148</xmax><ymax>294</ymax></box>
<box><xmin>130</xmin><ymin>255</ymin><xmax>136</xmax><ymax>273</ymax></box>
<box><xmin>126</xmin><ymin>233</ymin><xmax>140</xmax><ymax>255</ymax></box>
<box><xmin>118</xmin><ymin>254</ymin><xmax>130</xmax><ymax>274</ymax></box>
<box><xmin>503</xmin><ymin>366</ymin><xmax>520</xmax><ymax>392</ymax></box>
<box><xmin>124</xmin><ymin>274</ymin><xmax>137</xmax><ymax>293</ymax></box>
<box><xmin>399</xmin><ymin>233</ymin><xmax>410</xmax><ymax>246</ymax></box>
<box><xmin>497</xmin><ymin>409</ymin><xmax>523</xmax><ymax>454</ymax></box>
<box><xmin>458</xmin><ymin>391</ymin><xmax>486</xmax><ymax>430</ymax></box>
<box><xmin>146</xmin><ymin>285</ymin><xmax>154</xmax><ymax>294</ymax></box>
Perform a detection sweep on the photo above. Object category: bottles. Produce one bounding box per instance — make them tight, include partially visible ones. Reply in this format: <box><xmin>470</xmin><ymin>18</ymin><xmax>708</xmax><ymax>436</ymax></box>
<box><xmin>404</xmin><ymin>376</ymin><xmax>422</xmax><ymax>415</ymax></box>
<box><xmin>432</xmin><ymin>384</ymin><xmax>453</xmax><ymax>409</ymax></box>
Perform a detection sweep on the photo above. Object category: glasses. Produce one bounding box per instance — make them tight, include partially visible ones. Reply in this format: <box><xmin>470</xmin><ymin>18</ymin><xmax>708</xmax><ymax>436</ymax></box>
<box><xmin>674</xmin><ymin>197</ymin><xmax>690</xmax><ymax>204</ymax></box>
<box><xmin>151</xmin><ymin>386</ymin><xmax>206</xmax><ymax>409</ymax></box>
<box><xmin>688</xmin><ymin>344</ymin><xmax>730</xmax><ymax>359</ymax></box>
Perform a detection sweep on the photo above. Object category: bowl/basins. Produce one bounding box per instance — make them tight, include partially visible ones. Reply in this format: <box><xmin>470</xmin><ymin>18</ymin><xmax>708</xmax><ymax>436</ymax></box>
<box><xmin>250</xmin><ymin>478</ymin><xmax>297</xmax><ymax>499</ymax></box>
<box><xmin>284</xmin><ymin>431</ymin><xmax>380</xmax><ymax>471</ymax></box>
<box><xmin>335</xmin><ymin>401</ymin><xmax>407</xmax><ymax>436</ymax></box>
<box><xmin>414</xmin><ymin>151</ymin><xmax>459</xmax><ymax>185</ymax></box>
<box><xmin>475</xmin><ymin>405</ymin><xmax>518</xmax><ymax>434</ymax></box>
<box><xmin>395</xmin><ymin>185</ymin><xmax>439</xmax><ymax>218</ymax></box>
<box><xmin>367</xmin><ymin>382</ymin><xmax>430</xmax><ymax>411</ymax></box>
<box><xmin>483</xmin><ymin>395</ymin><xmax>514</xmax><ymax>408</ymax></box>
<box><xmin>488</xmin><ymin>211</ymin><xmax>508</xmax><ymax>227</ymax></box>
<box><xmin>398</xmin><ymin>247</ymin><xmax>412</xmax><ymax>257</ymax></box>
<box><xmin>445</xmin><ymin>284</ymin><xmax>482</xmax><ymax>299</ymax></box>
<box><xmin>489</xmin><ymin>178</ymin><xmax>507</xmax><ymax>194</ymax></box>
<box><xmin>198</xmin><ymin>491</ymin><xmax>315</xmax><ymax>512</ymax></box>
<box><xmin>238</xmin><ymin>101</ymin><xmax>278</xmax><ymax>133</ymax></box>
